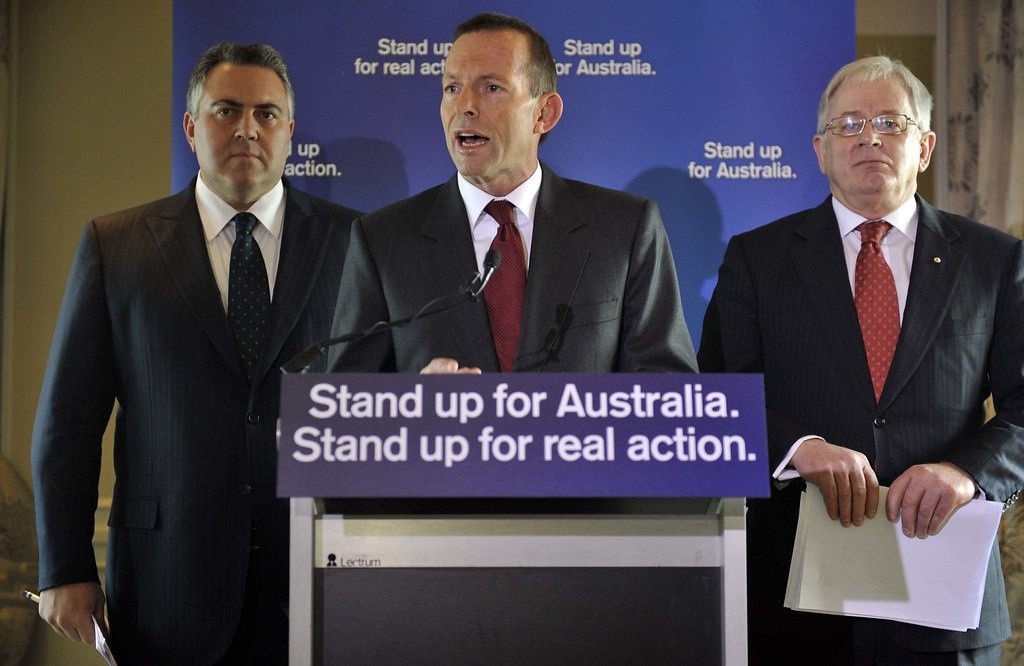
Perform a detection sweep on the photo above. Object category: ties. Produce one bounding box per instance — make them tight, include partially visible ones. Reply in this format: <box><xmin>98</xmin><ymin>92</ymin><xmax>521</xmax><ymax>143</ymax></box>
<box><xmin>855</xmin><ymin>222</ymin><xmax>902</xmax><ymax>405</ymax></box>
<box><xmin>228</xmin><ymin>213</ymin><xmax>274</xmax><ymax>376</ymax></box>
<box><xmin>483</xmin><ymin>200</ymin><xmax>530</xmax><ymax>373</ymax></box>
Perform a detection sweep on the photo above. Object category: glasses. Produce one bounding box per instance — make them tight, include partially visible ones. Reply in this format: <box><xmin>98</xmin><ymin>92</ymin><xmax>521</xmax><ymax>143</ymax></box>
<box><xmin>824</xmin><ymin>114</ymin><xmax>918</xmax><ymax>136</ymax></box>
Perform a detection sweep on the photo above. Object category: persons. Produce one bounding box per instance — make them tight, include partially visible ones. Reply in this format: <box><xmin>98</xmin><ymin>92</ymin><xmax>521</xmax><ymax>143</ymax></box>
<box><xmin>695</xmin><ymin>55</ymin><xmax>1024</xmax><ymax>665</ymax></box>
<box><xmin>30</xmin><ymin>43</ymin><xmax>363</xmax><ymax>666</ymax></box>
<box><xmin>327</xmin><ymin>13</ymin><xmax>711</xmax><ymax>515</ymax></box>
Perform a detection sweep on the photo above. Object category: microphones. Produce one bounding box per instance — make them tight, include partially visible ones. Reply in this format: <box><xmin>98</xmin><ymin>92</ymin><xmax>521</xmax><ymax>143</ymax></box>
<box><xmin>282</xmin><ymin>249</ymin><xmax>504</xmax><ymax>375</ymax></box>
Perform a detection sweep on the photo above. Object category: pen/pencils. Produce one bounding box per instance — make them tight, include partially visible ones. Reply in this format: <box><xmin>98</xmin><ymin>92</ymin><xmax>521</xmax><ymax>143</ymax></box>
<box><xmin>22</xmin><ymin>590</ymin><xmax>40</xmax><ymax>606</ymax></box>
